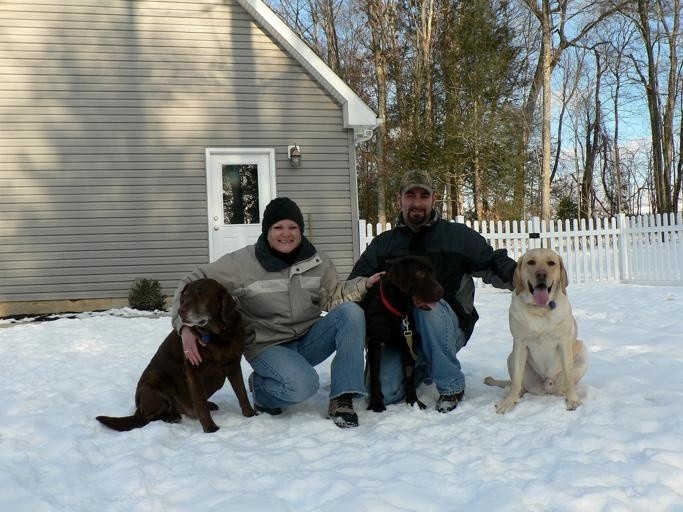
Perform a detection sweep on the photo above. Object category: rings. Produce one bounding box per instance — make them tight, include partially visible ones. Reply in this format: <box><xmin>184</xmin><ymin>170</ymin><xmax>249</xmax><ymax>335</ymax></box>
<box><xmin>184</xmin><ymin>351</ymin><xmax>188</xmax><ymax>354</ymax></box>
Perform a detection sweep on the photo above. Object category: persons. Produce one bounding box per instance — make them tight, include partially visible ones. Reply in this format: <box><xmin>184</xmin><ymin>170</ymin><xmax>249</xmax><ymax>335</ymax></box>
<box><xmin>169</xmin><ymin>194</ymin><xmax>387</xmax><ymax>430</ymax></box>
<box><xmin>341</xmin><ymin>169</ymin><xmax>519</xmax><ymax>413</ymax></box>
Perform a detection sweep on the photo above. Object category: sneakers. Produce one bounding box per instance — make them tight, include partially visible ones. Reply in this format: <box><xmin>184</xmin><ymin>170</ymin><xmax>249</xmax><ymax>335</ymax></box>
<box><xmin>248</xmin><ymin>372</ymin><xmax>283</xmax><ymax>415</ymax></box>
<box><xmin>327</xmin><ymin>394</ymin><xmax>358</xmax><ymax>428</ymax></box>
<box><xmin>436</xmin><ymin>391</ymin><xmax>465</xmax><ymax>412</ymax></box>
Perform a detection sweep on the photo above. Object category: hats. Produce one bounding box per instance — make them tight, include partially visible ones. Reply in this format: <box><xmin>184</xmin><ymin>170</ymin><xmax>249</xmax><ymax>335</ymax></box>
<box><xmin>399</xmin><ymin>170</ymin><xmax>435</xmax><ymax>196</ymax></box>
<box><xmin>263</xmin><ymin>197</ymin><xmax>305</xmax><ymax>242</ymax></box>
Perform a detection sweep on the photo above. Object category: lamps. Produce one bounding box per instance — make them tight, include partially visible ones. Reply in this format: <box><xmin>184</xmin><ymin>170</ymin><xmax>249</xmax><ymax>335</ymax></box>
<box><xmin>287</xmin><ymin>143</ymin><xmax>302</xmax><ymax>168</ymax></box>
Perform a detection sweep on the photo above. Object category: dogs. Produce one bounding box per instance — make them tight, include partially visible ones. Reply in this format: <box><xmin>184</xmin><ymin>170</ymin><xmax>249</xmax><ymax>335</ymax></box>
<box><xmin>484</xmin><ymin>248</ymin><xmax>588</xmax><ymax>414</ymax></box>
<box><xmin>357</xmin><ymin>256</ymin><xmax>445</xmax><ymax>413</ymax></box>
<box><xmin>95</xmin><ymin>277</ymin><xmax>260</xmax><ymax>433</ymax></box>
